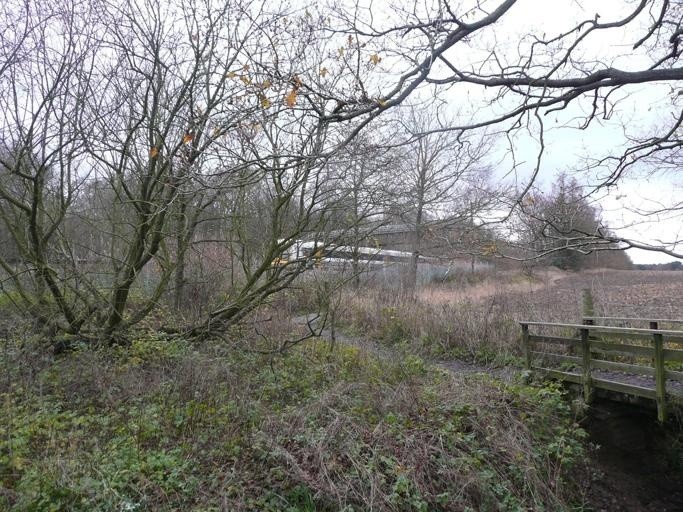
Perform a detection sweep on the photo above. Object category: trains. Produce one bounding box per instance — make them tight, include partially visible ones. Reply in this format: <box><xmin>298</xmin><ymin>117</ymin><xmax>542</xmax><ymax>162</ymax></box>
<box><xmin>288</xmin><ymin>240</ymin><xmax>435</xmax><ymax>266</ymax></box>
